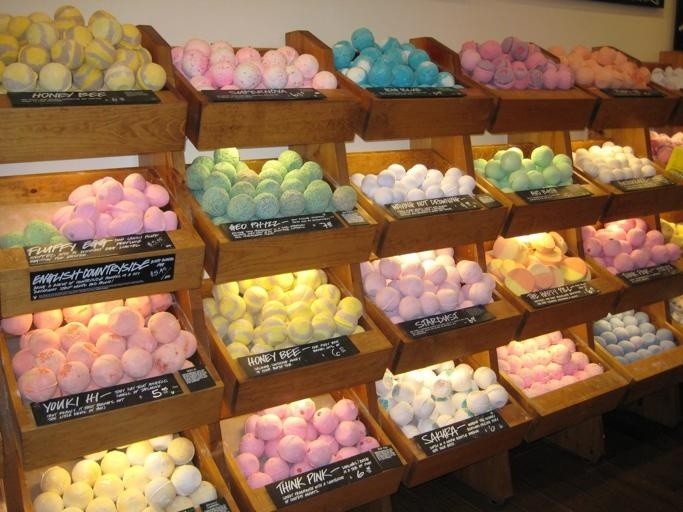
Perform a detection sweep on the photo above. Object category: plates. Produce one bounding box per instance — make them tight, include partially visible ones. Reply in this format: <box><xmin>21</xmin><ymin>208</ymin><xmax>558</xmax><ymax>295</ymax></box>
<box><xmin>0</xmin><ymin>22</ymin><xmax>683</xmax><ymax>511</ymax></box>
